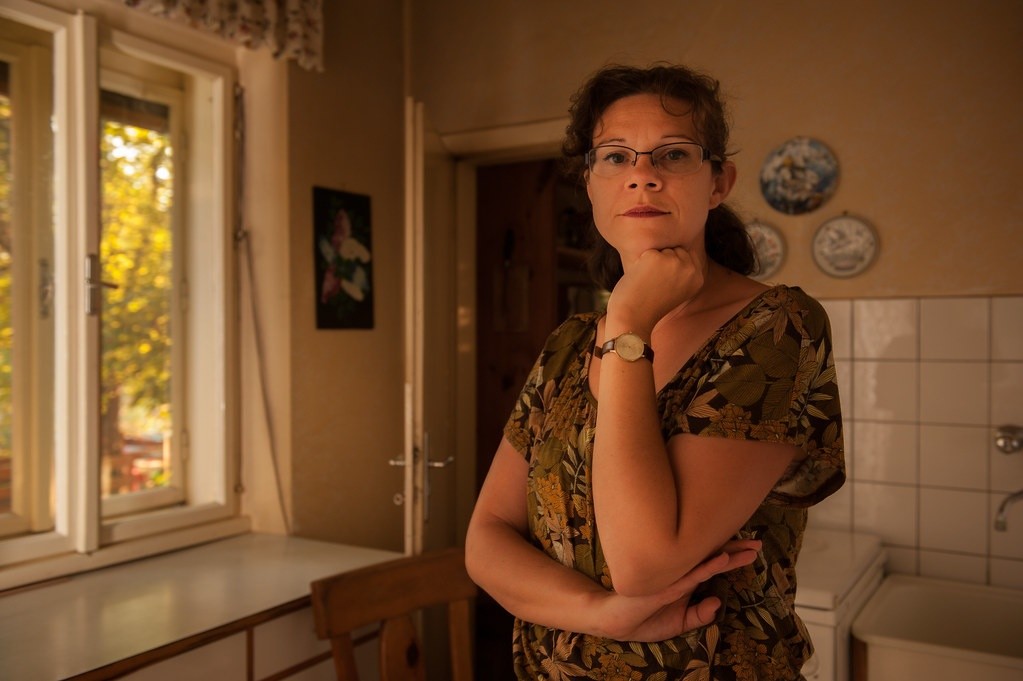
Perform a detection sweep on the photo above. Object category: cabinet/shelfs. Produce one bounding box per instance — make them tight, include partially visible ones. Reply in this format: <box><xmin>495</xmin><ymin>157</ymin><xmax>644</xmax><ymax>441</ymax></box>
<box><xmin>0</xmin><ymin>523</ymin><xmax>415</xmax><ymax>681</ymax></box>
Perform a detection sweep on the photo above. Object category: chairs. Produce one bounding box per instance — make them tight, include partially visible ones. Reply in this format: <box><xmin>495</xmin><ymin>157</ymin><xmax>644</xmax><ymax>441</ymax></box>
<box><xmin>310</xmin><ymin>543</ymin><xmax>477</xmax><ymax>681</ymax></box>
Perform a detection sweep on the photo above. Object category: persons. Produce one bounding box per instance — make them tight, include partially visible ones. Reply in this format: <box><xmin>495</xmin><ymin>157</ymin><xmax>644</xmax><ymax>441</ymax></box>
<box><xmin>465</xmin><ymin>64</ymin><xmax>846</xmax><ymax>681</ymax></box>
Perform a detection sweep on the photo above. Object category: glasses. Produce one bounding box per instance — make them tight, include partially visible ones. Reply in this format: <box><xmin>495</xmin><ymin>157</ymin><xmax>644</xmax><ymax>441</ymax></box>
<box><xmin>584</xmin><ymin>141</ymin><xmax>722</xmax><ymax>177</ymax></box>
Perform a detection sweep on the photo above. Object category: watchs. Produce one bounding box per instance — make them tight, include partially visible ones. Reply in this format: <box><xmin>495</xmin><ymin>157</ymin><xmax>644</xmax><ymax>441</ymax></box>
<box><xmin>594</xmin><ymin>332</ymin><xmax>654</xmax><ymax>364</ymax></box>
